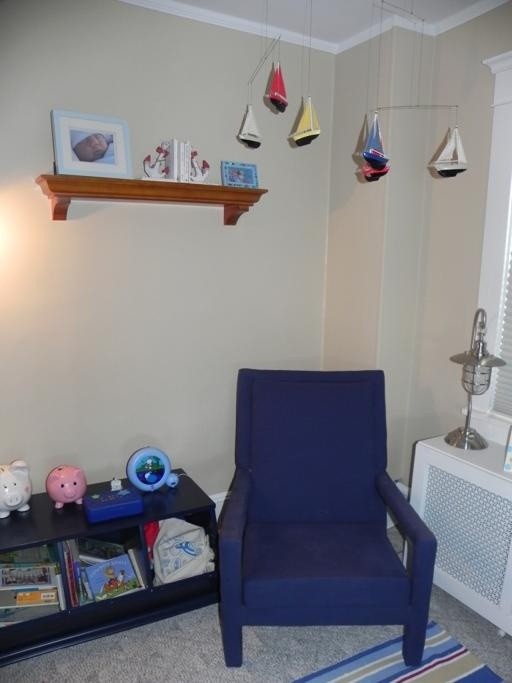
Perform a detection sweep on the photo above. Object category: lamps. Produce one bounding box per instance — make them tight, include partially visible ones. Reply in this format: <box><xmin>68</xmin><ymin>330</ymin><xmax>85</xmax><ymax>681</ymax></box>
<box><xmin>445</xmin><ymin>309</ymin><xmax>505</xmax><ymax>448</ymax></box>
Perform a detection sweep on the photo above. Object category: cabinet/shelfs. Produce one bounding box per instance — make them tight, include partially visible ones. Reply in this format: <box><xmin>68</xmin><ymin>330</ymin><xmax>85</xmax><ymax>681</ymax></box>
<box><xmin>402</xmin><ymin>435</ymin><xmax>512</xmax><ymax>638</ymax></box>
<box><xmin>0</xmin><ymin>469</ymin><xmax>219</xmax><ymax>668</ymax></box>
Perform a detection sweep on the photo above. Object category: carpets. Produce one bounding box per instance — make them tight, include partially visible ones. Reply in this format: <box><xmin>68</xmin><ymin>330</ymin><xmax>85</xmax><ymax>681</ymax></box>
<box><xmin>293</xmin><ymin>621</ymin><xmax>508</xmax><ymax>683</ymax></box>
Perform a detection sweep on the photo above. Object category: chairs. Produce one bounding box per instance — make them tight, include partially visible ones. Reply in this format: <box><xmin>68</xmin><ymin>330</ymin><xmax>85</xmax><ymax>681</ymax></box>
<box><xmin>218</xmin><ymin>369</ymin><xmax>437</xmax><ymax>667</ymax></box>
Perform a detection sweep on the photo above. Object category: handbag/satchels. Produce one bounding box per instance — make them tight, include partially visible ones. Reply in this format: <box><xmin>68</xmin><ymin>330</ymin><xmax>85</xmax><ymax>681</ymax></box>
<box><xmin>150</xmin><ymin>515</ymin><xmax>216</xmax><ymax>587</ymax></box>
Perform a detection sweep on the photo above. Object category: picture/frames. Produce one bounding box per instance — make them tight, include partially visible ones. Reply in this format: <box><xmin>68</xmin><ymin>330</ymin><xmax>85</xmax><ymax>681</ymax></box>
<box><xmin>50</xmin><ymin>110</ymin><xmax>134</xmax><ymax>180</ymax></box>
<box><xmin>220</xmin><ymin>160</ymin><xmax>259</xmax><ymax>189</ymax></box>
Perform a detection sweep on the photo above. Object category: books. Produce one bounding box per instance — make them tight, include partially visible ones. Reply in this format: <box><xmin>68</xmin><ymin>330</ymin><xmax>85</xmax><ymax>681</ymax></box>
<box><xmin>0</xmin><ymin>534</ymin><xmax>145</xmax><ymax>628</ymax></box>
